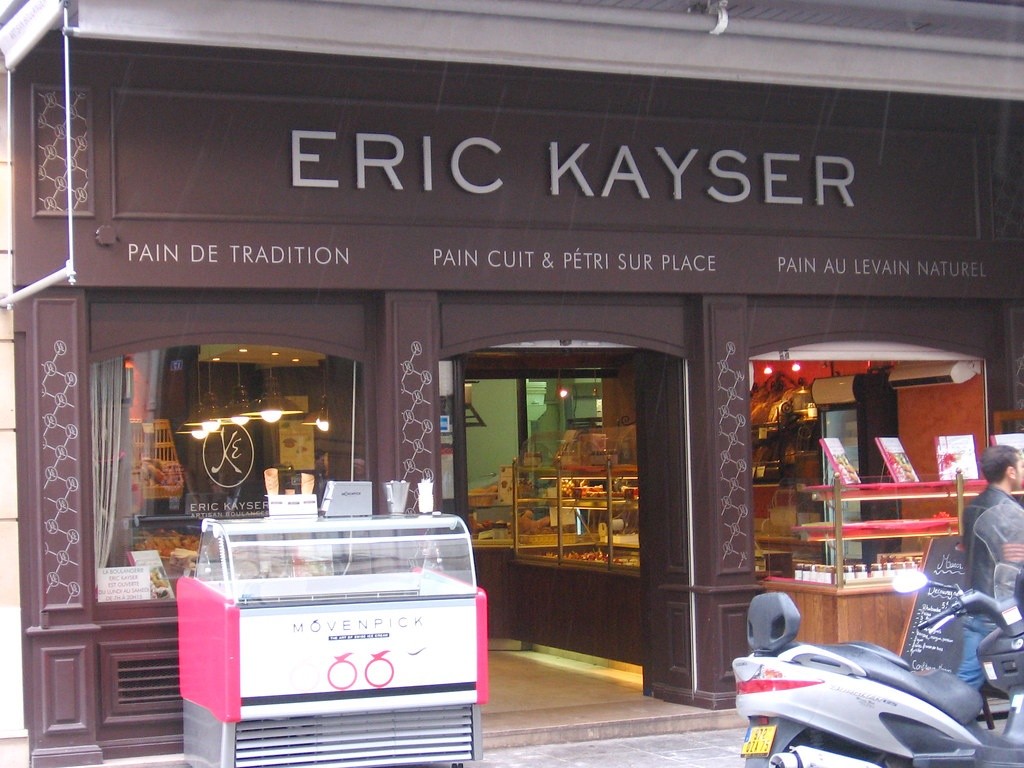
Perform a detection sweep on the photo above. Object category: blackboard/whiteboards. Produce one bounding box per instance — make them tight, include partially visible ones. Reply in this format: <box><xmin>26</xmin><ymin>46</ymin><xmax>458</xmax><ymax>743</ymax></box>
<box><xmin>896</xmin><ymin>534</ymin><xmax>971</xmax><ymax>679</ymax></box>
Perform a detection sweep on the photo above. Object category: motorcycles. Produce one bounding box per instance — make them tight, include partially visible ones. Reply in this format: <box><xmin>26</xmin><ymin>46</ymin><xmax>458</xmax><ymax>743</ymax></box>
<box><xmin>732</xmin><ymin>501</ymin><xmax>1024</xmax><ymax>768</ymax></box>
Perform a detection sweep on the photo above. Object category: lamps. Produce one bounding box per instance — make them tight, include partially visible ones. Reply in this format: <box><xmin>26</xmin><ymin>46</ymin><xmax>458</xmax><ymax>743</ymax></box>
<box><xmin>174</xmin><ymin>344</ymin><xmax>333</xmax><ymax>440</ymax></box>
<box><xmin>949</xmin><ymin>361</ymin><xmax>981</xmax><ymax>383</ymax></box>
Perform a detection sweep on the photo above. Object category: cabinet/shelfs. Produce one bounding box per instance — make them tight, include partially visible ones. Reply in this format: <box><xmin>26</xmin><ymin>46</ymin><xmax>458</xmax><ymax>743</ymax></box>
<box><xmin>512</xmin><ymin>458</ymin><xmax>640</xmax><ymax>577</ymax></box>
<box><xmin>750</xmin><ymin>417</ymin><xmax>823</xmax><ymax>546</ymax></box>
<box><xmin>788</xmin><ymin>478</ymin><xmax>994</xmax><ymax>541</ymax></box>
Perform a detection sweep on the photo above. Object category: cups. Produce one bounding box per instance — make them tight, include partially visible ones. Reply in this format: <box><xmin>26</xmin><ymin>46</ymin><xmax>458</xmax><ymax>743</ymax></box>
<box><xmin>383</xmin><ymin>483</ymin><xmax>411</xmax><ymax>515</ymax></box>
<box><xmin>418</xmin><ymin>494</ymin><xmax>434</xmax><ymax>514</ymax></box>
<box><xmin>793</xmin><ymin>561</ymin><xmax>917</xmax><ymax>584</ymax></box>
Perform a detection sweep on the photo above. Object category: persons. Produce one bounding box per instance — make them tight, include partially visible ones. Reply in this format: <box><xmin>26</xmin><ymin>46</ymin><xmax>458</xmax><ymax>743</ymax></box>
<box><xmin>955</xmin><ymin>444</ymin><xmax>1024</xmax><ymax>693</ymax></box>
